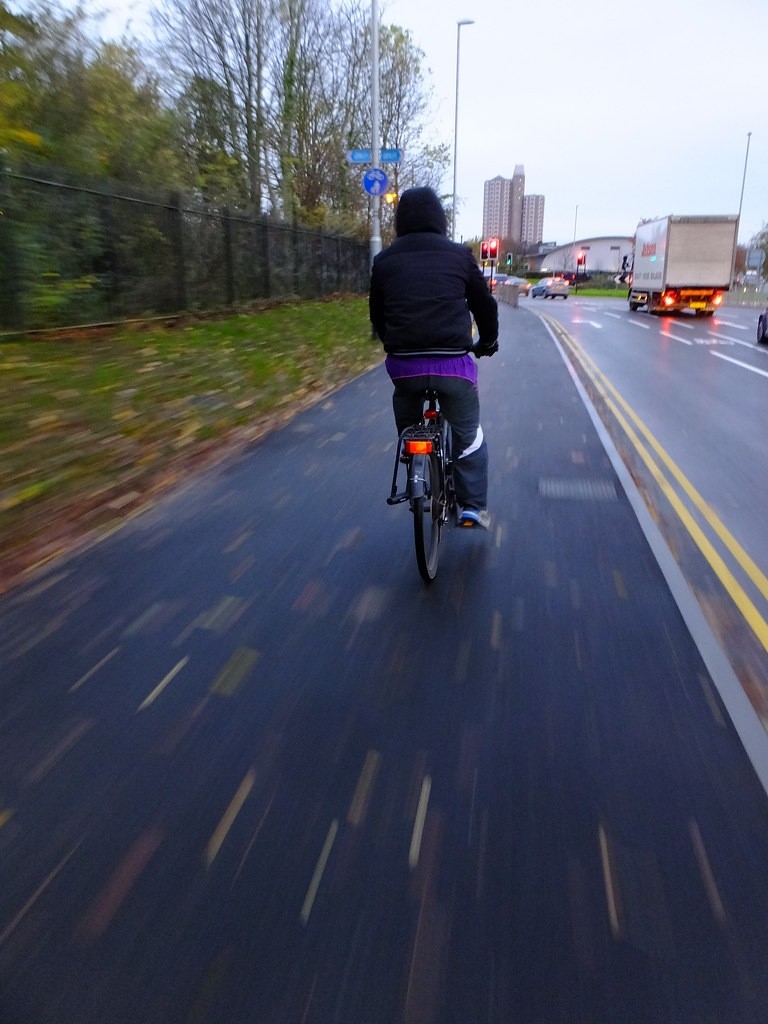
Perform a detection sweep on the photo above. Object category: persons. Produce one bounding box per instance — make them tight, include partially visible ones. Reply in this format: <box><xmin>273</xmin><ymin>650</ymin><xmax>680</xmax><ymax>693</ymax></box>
<box><xmin>369</xmin><ymin>186</ymin><xmax>499</xmax><ymax>530</ymax></box>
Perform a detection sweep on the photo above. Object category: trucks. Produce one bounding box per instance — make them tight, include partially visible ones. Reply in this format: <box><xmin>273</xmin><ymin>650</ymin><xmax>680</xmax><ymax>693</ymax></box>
<box><xmin>621</xmin><ymin>214</ymin><xmax>740</xmax><ymax>316</ymax></box>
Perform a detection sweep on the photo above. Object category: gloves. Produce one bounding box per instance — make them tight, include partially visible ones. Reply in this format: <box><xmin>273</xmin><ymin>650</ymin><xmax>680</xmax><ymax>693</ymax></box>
<box><xmin>474</xmin><ymin>340</ymin><xmax>499</xmax><ymax>358</ymax></box>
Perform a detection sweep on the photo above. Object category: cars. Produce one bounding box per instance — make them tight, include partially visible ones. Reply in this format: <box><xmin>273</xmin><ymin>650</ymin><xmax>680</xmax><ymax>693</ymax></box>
<box><xmin>757</xmin><ymin>306</ymin><xmax>768</xmax><ymax>342</ymax></box>
<box><xmin>561</xmin><ymin>272</ymin><xmax>590</xmax><ymax>286</ymax></box>
<box><xmin>531</xmin><ymin>277</ymin><xmax>569</xmax><ymax>299</ymax></box>
<box><xmin>736</xmin><ymin>274</ymin><xmax>763</xmax><ymax>287</ymax></box>
<box><xmin>487</xmin><ymin>274</ymin><xmax>509</xmax><ymax>293</ymax></box>
<box><xmin>504</xmin><ymin>279</ymin><xmax>530</xmax><ymax>296</ymax></box>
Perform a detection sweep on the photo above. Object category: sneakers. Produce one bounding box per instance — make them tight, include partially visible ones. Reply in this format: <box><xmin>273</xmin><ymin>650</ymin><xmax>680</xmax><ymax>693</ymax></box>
<box><xmin>461</xmin><ymin>505</ymin><xmax>492</xmax><ymax>530</ymax></box>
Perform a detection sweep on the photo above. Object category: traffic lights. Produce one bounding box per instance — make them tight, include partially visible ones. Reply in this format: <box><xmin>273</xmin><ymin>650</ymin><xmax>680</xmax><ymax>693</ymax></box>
<box><xmin>480</xmin><ymin>242</ymin><xmax>489</xmax><ymax>261</ymax></box>
<box><xmin>507</xmin><ymin>254</ymin><xmax>512</xmax><ymax>266</ymax></box>
<box><xmin>578</xmin><ymin>252</ymin><xmax>583</xmax><ymax>265</ymax></box>
<box><xmin>489</xmin><ymin>240</ymin><xmax>499</xmax><ymax>260</ymax></box>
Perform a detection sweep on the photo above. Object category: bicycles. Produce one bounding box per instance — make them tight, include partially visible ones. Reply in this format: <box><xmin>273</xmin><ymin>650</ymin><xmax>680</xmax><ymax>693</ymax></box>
<box><xmin>402</xmin><ymin>343</ymin><xmax>501</xmax><ymax>583</ymax></box>
